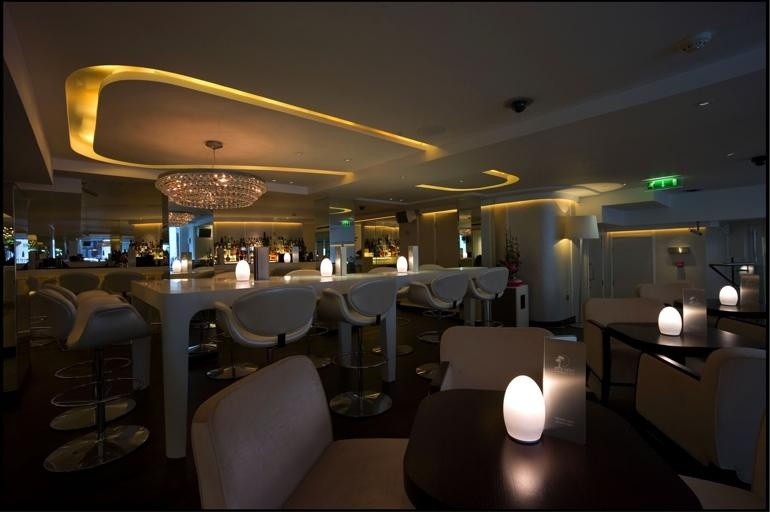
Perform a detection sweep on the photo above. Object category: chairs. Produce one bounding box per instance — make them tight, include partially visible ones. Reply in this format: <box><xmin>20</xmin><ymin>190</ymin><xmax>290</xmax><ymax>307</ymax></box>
<box><xmin>190</xmin><ymin>355</ymin><xmax>415</xmax><ymax>512</ymax></box>
<box><xmin>431</xmin><ymin>326</ymin><xmax>561</xmax><ymax>393</ymax></box>
<box><xmin>583</xmin><ymin>276</ymin><xmax>766</xmax><ymax>512</ymax></box>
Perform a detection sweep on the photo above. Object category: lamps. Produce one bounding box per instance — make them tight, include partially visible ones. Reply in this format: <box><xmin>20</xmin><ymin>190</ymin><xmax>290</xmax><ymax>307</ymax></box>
<box><xmin>167</xmin><ymin>210</ymin><xmax>196</xmax><ymax>227</ymax></box>
<box><xmin>154</xmin><ymin>139</ymin><xmax>268</xmax><ymax>212</ymax></box>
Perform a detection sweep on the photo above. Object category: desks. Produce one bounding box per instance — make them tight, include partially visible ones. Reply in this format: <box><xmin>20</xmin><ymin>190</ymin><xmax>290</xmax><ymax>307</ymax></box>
<box><xmin>404</xmin><ymin>390</ymin><xmax>702</xmax><ymax>512</ymax></box>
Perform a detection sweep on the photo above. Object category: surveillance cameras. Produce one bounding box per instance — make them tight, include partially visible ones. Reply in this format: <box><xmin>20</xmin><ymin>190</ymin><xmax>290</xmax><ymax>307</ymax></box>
<box><xmin>511</xmin><ymin>98</ymin><xmax>528</xmax><ymax>113</ymax></box>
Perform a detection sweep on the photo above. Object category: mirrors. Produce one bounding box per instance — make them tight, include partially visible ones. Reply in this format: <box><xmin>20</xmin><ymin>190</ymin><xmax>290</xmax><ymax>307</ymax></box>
<box><xmin>456</xmin><ymin>196</ymin><xmax>484</xmax><ymax>324</ymax></box>
<box><xmin>315</xmin><ymin>200</ymin><xmax>329</xmax><ymax>265</ymax></box>
<box><xmin>12</xmin><ymin>184</ymin><xmax>33</xmax><ymax>390</ymax></box>
<box><xmin>1</xmin><ymin>179</ymin><xmax>18</xmax><ymax>394</ymax></box>
<box><xmin>161</xmin><ymin>192</ymin><xmax>214</xmax><ymax>279</ymax></box>
<box><xmin>329</xmin><ymin>200</ymin><xmax>355</xmax><ymax>265</ymax></box>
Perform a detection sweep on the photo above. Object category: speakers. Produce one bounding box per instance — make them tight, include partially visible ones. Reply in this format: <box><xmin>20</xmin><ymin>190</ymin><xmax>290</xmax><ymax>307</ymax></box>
<box><xmin>83</xmin><ymin>240</ymin><xmax>91</xmax><ymax>246</ymax></box>
<box><xmin>197</xmin><ymin>227</ymin><xmax>212</xmax><ymax>239</ymax></box>
<box><xmin>396</xmin><ymin>209</ymin><xmax>416</xmax><ymax>224</ymax></box>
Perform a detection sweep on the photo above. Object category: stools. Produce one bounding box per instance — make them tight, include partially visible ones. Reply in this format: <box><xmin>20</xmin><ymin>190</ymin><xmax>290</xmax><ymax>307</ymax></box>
<box><xmin>3</xmin><ymin>261</ymin><xmax>510</xmax><ymax>472</ymax></box>
